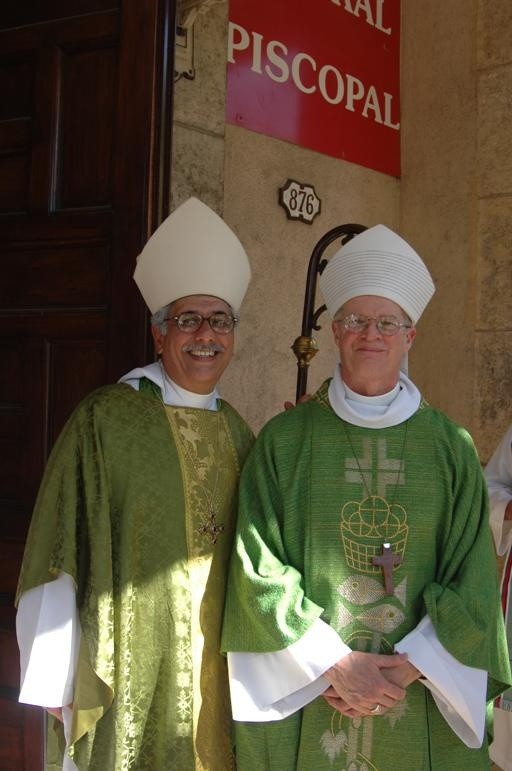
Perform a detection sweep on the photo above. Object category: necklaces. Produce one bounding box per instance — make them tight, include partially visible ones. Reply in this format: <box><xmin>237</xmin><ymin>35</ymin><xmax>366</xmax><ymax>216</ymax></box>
<box><xmin>342</xmin><ymin>420</ymin><xmax>409</xmax><ymax>596</ymax></box>
<box><xmin>167</xmin><ymin>404</ymin><xmax>225</xmax><ymax>545</ymax></box>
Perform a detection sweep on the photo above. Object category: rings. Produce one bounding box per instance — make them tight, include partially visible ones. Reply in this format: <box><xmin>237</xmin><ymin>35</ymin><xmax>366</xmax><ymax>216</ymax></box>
<box><xmin>370</xmin><ymin>705</ymin><xmax>385</xmax><ymax>715</ymax></box>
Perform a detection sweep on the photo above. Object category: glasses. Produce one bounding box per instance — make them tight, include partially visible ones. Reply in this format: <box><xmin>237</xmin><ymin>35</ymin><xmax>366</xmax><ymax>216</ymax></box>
<box><xmin>159</xmin><ymin>310</ymin><xmax>238</xmax><ymax>337</ymax></box>
<box><xmin>331</xmin><ymin>312</ymin><xmax>411</xmax><ymax>338</ymax></box>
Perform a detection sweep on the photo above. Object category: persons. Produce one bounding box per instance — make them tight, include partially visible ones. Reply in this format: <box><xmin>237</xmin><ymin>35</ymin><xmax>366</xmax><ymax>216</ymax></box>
<box><xmin>482</xmin><ymin>420</ymin><xmax>512</xmax><ymax>771</ymax></box>
<box><xmin>13</xmin><ymin>293</ymin><xmax>313</xmax><ymax>771</ymax></box>
<box><xmin>219</xmin><ymin>293</ymin><xmax>512</xmax><ymax>771</ymax></box>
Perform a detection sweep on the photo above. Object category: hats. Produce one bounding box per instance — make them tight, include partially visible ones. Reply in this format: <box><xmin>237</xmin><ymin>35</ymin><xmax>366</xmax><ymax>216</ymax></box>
<box><xmin>131</xmin><ymin>195</ymin><xmax>252</xmax><ymax>316</ymax></box>
<box><xmin>315</xmin><ymin>222</ymin><xmax>436</xmax><ymax>329</ymax></box>
